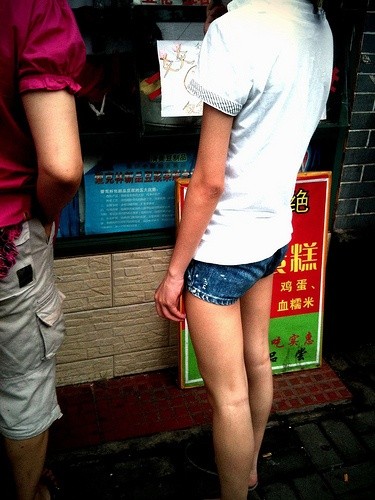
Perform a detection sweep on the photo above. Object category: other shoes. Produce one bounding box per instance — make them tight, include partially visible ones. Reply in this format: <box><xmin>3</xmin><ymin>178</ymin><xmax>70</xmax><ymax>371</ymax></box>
<box><xmin>187</xmin><ymin>442</ymin><xmax>258</xmax><ymax>491</ymax></box>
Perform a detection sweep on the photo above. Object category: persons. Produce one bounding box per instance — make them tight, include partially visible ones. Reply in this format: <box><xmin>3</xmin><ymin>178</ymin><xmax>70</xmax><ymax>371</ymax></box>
<box><xmin>154</xmin><ymin>0</ymin><xmax>334</xmax><ymax>500</ymax></box>
<box><xmin>0</xmin><ymin>0</ymin><xmax>89</xmax><ymax>500</ymax></box>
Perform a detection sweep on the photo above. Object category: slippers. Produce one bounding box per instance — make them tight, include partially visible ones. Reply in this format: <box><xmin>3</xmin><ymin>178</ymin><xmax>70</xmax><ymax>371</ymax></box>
<box><xmin>40</xmin><ymin>463</ymin><xmax>68</xmax><ymax>500</ymax></box>
